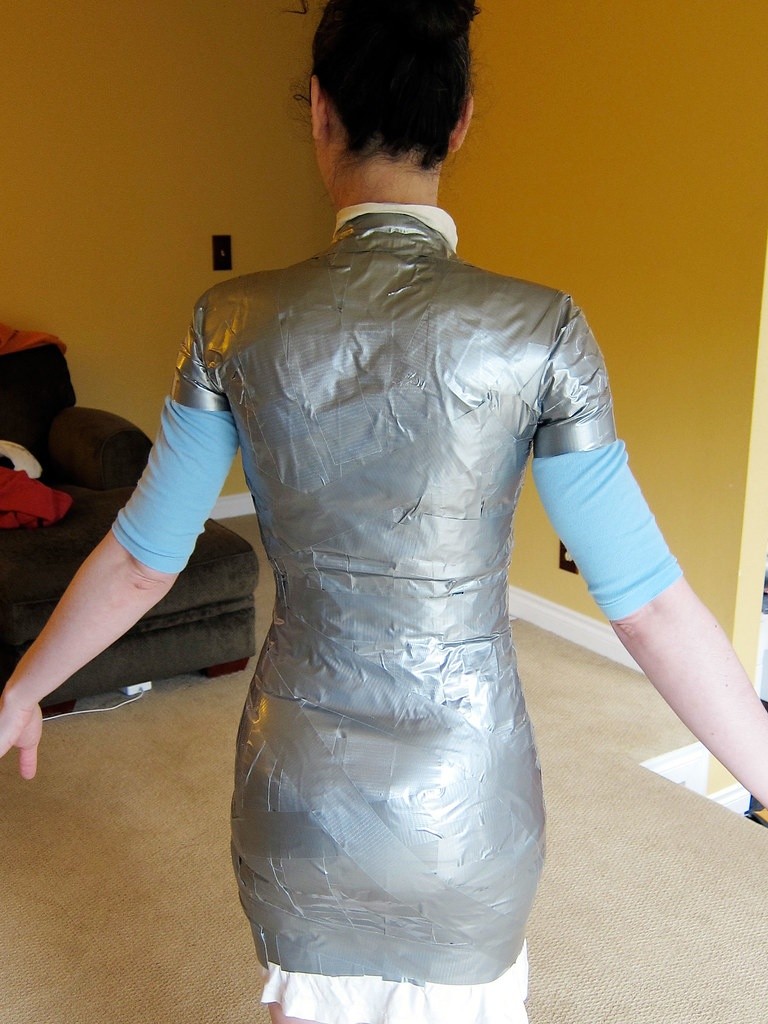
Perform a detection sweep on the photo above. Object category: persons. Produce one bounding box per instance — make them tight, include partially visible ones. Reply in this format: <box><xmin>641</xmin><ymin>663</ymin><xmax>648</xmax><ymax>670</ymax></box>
<box><xmin>0</xmin><ymin>0</ymin><xmax>767</xmax><ymax>1024</ymax></box>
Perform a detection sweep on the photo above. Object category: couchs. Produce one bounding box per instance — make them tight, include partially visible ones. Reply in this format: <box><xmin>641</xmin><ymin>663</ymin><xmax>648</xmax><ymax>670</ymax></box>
<box><xmin>0</xmin><ymin>344</ymin><xmax>259</xmax><ymax>718</ymax></box>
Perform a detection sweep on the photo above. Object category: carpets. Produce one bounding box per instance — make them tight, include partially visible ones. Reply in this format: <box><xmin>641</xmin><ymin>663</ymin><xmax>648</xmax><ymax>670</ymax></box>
<box><xmin>638</xmin><ymin>739</ymin><xmax>768</xmax><ymax>828</ymax></box>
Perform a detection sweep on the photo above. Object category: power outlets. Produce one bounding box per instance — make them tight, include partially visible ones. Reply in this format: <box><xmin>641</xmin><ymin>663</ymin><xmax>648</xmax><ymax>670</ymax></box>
<box><xmin>559</xmin><ymin>541</ymin><xmax>579</xmax><ymax>575</ymax></box>
